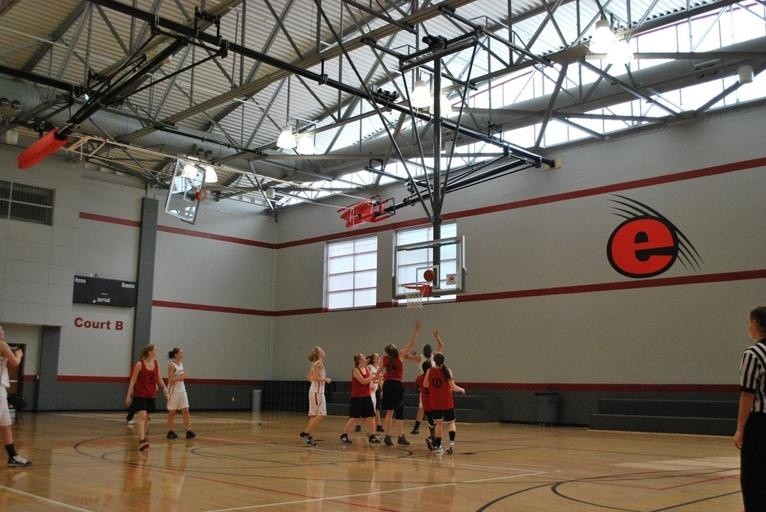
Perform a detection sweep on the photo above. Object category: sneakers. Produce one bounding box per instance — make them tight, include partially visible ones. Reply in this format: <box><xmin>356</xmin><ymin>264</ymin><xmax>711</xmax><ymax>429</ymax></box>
<box><xmin>166</xmin><ymin>431</ymin><xmax>178</xmax><ymax>439</ymax></box>
<box><xmin>354</xmin><ymin>425</ymin><xmax>362</xmax><ymax>432</ymax></box>
<box><xmin>383</xmin><ymin>433</ymin><xmax>394</xmax><ymax>446</ymax></box>
<box><xmin>6</xmin><ymin>454</ymin><xmax>33</xmax><ymax>468</ymax></box>
<box><xmin>445</xmin><ymin>442</ymin><xmax>456</xmax><ymax>455</ymax></box>
<box><xmin>435</xmin><ymin>444</ymin><xmax>444</xmax><ymax>453</ymax></box>
<box><xmin>367</xmin><ymin>432</ymin><xmax>383</xmax><ymax>444</ymax></box>
<box><xmin>298</xmin><ymin>431</ymin><xmax>318</xmax><ymax>447</ymax></box>
<box><xmin>397</xmin><ymin>434</ymin><xmax>411</xmax><ymax>445</ymax></box>
<box><xmin>126</xmin><ymin>419</ymin><xmax>135</xmax><ymax>424</ymax></box>
<box><xmin>375</xmin><ymin>424</ymin><xmax>384</xmax><ymax>431</ymax></box>
<box><xmin>339</xmin><ymin>433</ymin><xmax>353</xmax><ymax>444</ymax></box>
<box><xmin>411</xmin><ymin>429</ymin><xmax>420</xmax><ymax>434</ymax></box>
<box><xmin>139</xmin><ymin>439</ymin><xmax>150</xmax><ymax>452</ymax></box>
<box><xmin>184</xmin><ymin>430</ymin><xmax>200</xmax><ymax>440</ymax></box>
<box><xmin>425</xmin><ymin>435</ymin><xmax>437</xmax><ymax>451</ymax></box>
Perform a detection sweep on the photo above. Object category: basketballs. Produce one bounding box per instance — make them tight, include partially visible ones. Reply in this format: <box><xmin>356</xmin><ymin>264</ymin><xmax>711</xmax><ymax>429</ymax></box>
<box><xmin>424</xmin><ymin>270</ymin><xmax>435</xmax><ymax>281</ymax></box>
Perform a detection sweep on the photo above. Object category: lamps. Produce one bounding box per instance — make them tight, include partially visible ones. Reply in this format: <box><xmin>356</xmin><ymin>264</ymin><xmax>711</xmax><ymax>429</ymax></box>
<box><xmin>410</xmin><ymin>80</ymin><xmax>431</xmax><ymax>108</ymax></box>
<box><xmin>276</xmin><ymin>126</ymin><xmax>315</xmax><ymax>154</ymax></box>
<box><xmin>589</xmin><ymin>20</ymin><xmax>619</xmax><ymax>54</ymax></box>
<box><xmin>606</xmin><ymin>35</ymin><xmax>634</xmax><ymax>65</ymax></box>
<box><xmin>181</xmin><ymin>162</ymin><xmax>218</xmax><ymax>183</ymax></box>
<box><xmin>429</xmin><ymin>94</ymin><xmax>452</xmax><ymax>115</ymax></box>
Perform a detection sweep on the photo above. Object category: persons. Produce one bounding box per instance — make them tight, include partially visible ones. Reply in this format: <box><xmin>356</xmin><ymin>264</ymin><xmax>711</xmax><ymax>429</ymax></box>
<box><xmin>0</xmin><ymin>324</ymin><xmax>32</xmax><ymax>467</ymax></box>
<box><xmin>124</xmin><ymin>343</ymin><xmax>171</xmax><ymax>450</ymax></box>
<box><xmin>123</xmin><ymin>422</ymin><xmax>196</xmax><ymax>511</ymax></box>
<box><xmin>0</xmin><ymin>464</ymin><xmax>30</xmax><ymax>504</ymax></box>
<box><xmin>339</xmin><ymin>318</ymin><xmax>466</xmax><ymax>457</ymax></box>
<box><xmin>165</xmin><ymin>347</ymin><xmax>200</xmax><ymax>439</ymax></box>
<box><xmin>298</xmin><ymin>344</ymin><xmax>333</xmax><ymax>447</ymax></box>
<box><xmin>125</xmin><ymin>350</ymin><xmax>159</xmax><ymax>431</ymax></box>
<box><xmin>733</xmin><ymin>304</ymin><xmax>766</xmax><ymax>511</ymax></box>
<box><xmin>336</xmin><ymin>439</ymin><xmax>459</xmax><ymax>511</ymax></box>
<box><xmin>296</xmin><ymin>444</ymin><xmax>325</xmax><ymax>510</ymax></box>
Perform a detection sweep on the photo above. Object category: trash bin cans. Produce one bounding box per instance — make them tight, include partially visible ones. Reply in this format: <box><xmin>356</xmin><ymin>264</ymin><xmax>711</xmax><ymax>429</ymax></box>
<box><xmin>252</xmin><ymin>389</ymin><xmax>262</xmax><ymax>412</ymax></box>
<box><xmin>535</xmin><ymin>392</ymin><xmax>559</xmax><ymax>426</ymax></box>
